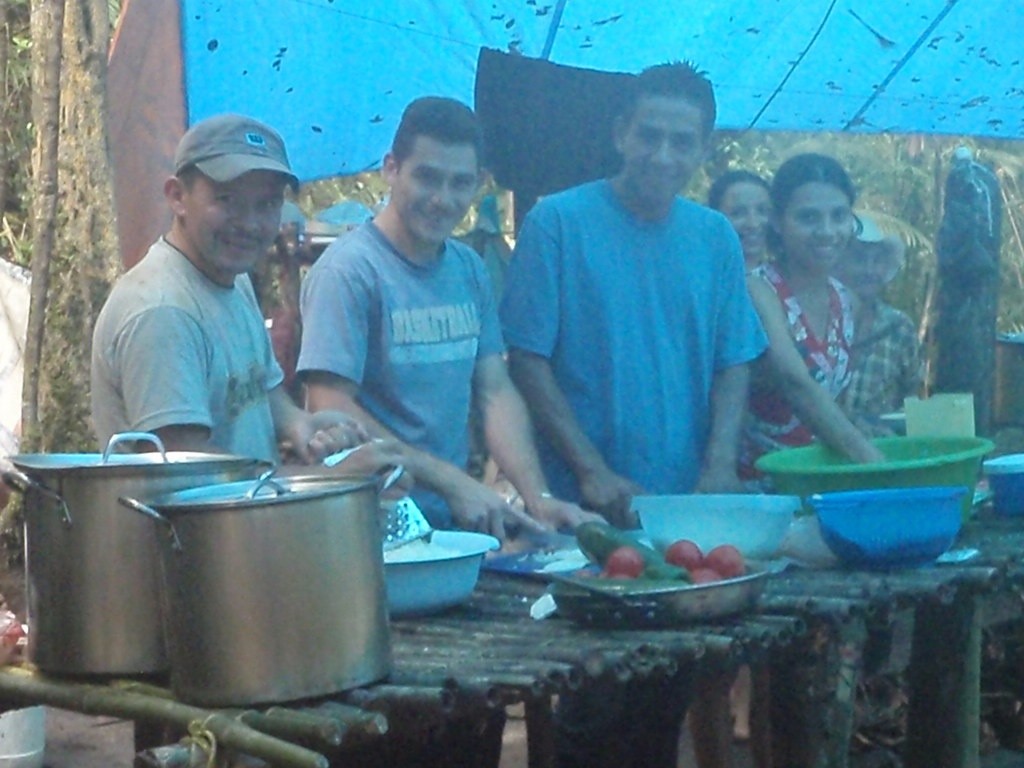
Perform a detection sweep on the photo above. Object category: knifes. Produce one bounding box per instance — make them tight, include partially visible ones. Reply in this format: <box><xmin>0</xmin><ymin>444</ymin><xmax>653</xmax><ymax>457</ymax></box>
<box><xmin>507</xmin><ymin>524</ymin><xmax>580</xmax><ymax>555</ymax></box>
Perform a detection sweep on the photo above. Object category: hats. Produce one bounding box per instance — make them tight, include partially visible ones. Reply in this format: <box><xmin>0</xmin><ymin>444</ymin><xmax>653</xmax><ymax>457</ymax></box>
<box><xmin>173</xmin><ymin>114</ymin><xmax>302</xmax><ymax>194</ymax></box>
<box><xmin>855</xmin><ymin>216</ymin><xmax>905</xmax><ymax>282</ymax></box>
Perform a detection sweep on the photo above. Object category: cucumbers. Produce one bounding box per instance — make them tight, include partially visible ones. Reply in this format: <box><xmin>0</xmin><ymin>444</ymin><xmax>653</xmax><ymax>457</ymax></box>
<box><xmin>576</xmin><ymin>520</ymin><xmax>692</xmax><ymax>582</ymax></box>
<box><xmin>562</xmin><ymin>575</ymin><xmax>690</xmax><ymax>591</ymax></box>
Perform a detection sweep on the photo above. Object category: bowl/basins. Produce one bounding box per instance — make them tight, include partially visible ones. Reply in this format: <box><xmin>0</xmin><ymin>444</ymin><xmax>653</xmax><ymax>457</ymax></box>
<box><xmin>381</xmin><ymin>530</ymin><xmax>500</xmax><ymax>616</ymax></box>
<box><xmin>808</xmin><ymin>486</ymin><xmax>968</xmax><ymax>570</ymax></box>
<box><xmin>753</xmin><ymin>434</ymin><xmax>995</xmax><ymax>524</ymax></box>
<box><xmin>629</xmin><ymin>495</ymin><xmax>802</xmax><ymax>559</ymax></box>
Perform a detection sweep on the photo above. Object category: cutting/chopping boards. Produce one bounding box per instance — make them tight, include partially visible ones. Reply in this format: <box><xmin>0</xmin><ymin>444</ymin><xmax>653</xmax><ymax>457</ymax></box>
<box><xmin>484</xmin><ymin>549</ymin><xmax>556</xmax><ymax>584</ymax></box>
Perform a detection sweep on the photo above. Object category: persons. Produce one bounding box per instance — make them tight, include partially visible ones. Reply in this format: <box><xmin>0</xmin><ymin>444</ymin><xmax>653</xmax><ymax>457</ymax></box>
<box><xmin>497</xmin><ymin>57</ymin><xmax>770</xmax><ymax>768</ymax></box>
<box><xmin>706</xmin><ymin>169</ymin><xmax>774</xmax><ymax>278</ymax></box>
<box><xmin>246</xmin><ymin>173</ymin><xmax>306</xmax><ymax>371</ymax></box>
<box><xmin>692</xmin><ymin>152</ymin><xmax>864</xmax><ymax>768</ymax></box>
<box><xmin>295</xmin><ymin>96</ymin><xmax>609</xmax><ymax>768</ymax></box>
<box><xmin>835</xmin><ymin>216</ymin><xmax>927</xmax><ymax>744</ymax></box>
<box><xmin>91</xmin><ymin>114</ymin><xmax>412</xmax><ymax>499</ymax></box>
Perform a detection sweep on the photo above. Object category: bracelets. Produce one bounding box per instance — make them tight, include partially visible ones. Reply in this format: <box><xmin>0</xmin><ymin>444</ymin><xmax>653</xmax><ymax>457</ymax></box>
<box><xmin>535</xmin><ymin>492</ymin><xmax>557</xmax><ymax>501</ymax></box>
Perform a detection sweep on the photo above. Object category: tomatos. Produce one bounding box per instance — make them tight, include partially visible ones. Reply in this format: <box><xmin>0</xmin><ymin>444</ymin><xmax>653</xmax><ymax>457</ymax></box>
<box><xmin>666</xmin><ymin>539</ymin><xmax>745</xmax><ymax>585</ymax></box>
<box><xmin>576</xmin><ymin>547</ymin><xmax>642</xmax><ymax>581</ymax></box>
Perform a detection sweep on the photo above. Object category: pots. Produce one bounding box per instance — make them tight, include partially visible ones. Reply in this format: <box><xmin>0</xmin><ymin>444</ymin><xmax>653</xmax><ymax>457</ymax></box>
<box><xmin>547</xmin><ymin>562</ymin><xmax>767</xmax><ymax>628</ymax></box>
<box><xmin>3</xmin><ymin>432</ymin><xmax>273</xmax><ymax>676</ymax></box>
<box><xmin>119</xmin><ymin>462</ymin><xmax>404</xmax><ymax>708</ymax></box>
